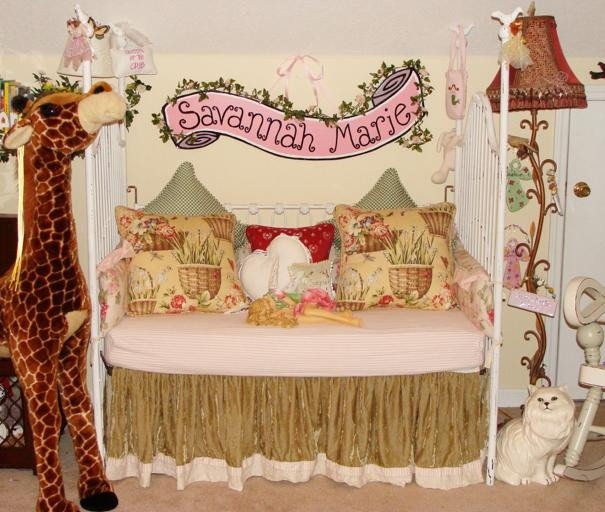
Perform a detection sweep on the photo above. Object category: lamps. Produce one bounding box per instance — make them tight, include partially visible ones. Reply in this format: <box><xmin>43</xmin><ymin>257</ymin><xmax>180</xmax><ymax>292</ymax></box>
<box><xmin>484</xmin><ymin>14</ymin><xmax>593</xmax><ymax>396</ymax></box>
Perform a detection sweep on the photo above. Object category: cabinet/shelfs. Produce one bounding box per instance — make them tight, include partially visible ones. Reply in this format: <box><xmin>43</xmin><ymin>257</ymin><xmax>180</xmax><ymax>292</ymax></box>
<box><xmin>0</xmin><ymin>347</ymin><xmax>41</xmax><ymax>477</ymax></box>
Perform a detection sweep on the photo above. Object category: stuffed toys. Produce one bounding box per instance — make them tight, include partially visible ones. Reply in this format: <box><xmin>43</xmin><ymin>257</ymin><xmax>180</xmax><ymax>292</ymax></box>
<box><xmin>0</xmin><ymin>81</ymin><xmax>130</xmax><ymax>510</ymax></box>
<box><xmin>429</xmin><ymin>128</ymin><xmax>465</xmax><ymax>185</ymax></box>
<box><xmin>247</xmin><ymin>284</ymin><xmax>363</xmax><ymax>329</ymax></box>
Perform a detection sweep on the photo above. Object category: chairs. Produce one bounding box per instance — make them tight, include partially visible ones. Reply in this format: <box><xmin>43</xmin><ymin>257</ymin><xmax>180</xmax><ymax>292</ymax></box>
<box><xmin>551</xmin><ymin>273</ymin><xmax>605</xmax><ymax>483</ymax></box>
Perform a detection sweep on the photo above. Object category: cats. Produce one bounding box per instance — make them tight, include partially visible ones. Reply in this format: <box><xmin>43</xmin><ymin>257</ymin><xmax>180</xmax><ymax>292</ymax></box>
<box><xmin>494</xmin><ymin>383</ymin><xmax>576</xmax><ymax>485</ymax></box>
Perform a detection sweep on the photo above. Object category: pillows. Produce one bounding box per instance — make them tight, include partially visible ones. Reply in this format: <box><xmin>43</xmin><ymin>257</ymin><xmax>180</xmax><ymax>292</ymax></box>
<box><xmin>126</xmin><ymin>162</ymin><xmax>249</xmax><ymax>251</ymax></box>
<box><xmin>240</xmin><ymin>221</ymin><xmax>336</xmax><ymax>266</ymax></box>
<box><xmin>109</xmin><ymin>205</ymin><xmax>255</xmax><ymax>317</ymax></box>
<box><xmin>96</xmin><ymin>242</ymin><xmax>130</xmax><ymax>340</ymax></box>
<box><xmin>331</xmin><ymin>199</ymin><xmax>457</xmax><ymax>313</ymax></box>
<box><xmin>454</xmin><ymin>238</ymin><xmax>498</xmax><ymax>340</ymax></box>
<box><xmin>287</xmin><ymin>258</ymin><xmax>335</xmax><ymax>299</ymax></box>
<box><xmin>318</xmin><ymin>166</ymin><xmax>420</xmax><ymax>257</ymax></box>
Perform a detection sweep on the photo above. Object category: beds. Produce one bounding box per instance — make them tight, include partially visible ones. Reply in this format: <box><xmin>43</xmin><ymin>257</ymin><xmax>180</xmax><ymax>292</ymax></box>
<box><xmin>61</xmin><ymin>2</ymin><xmax>520</xmax><ymax>489</ymax></box>
